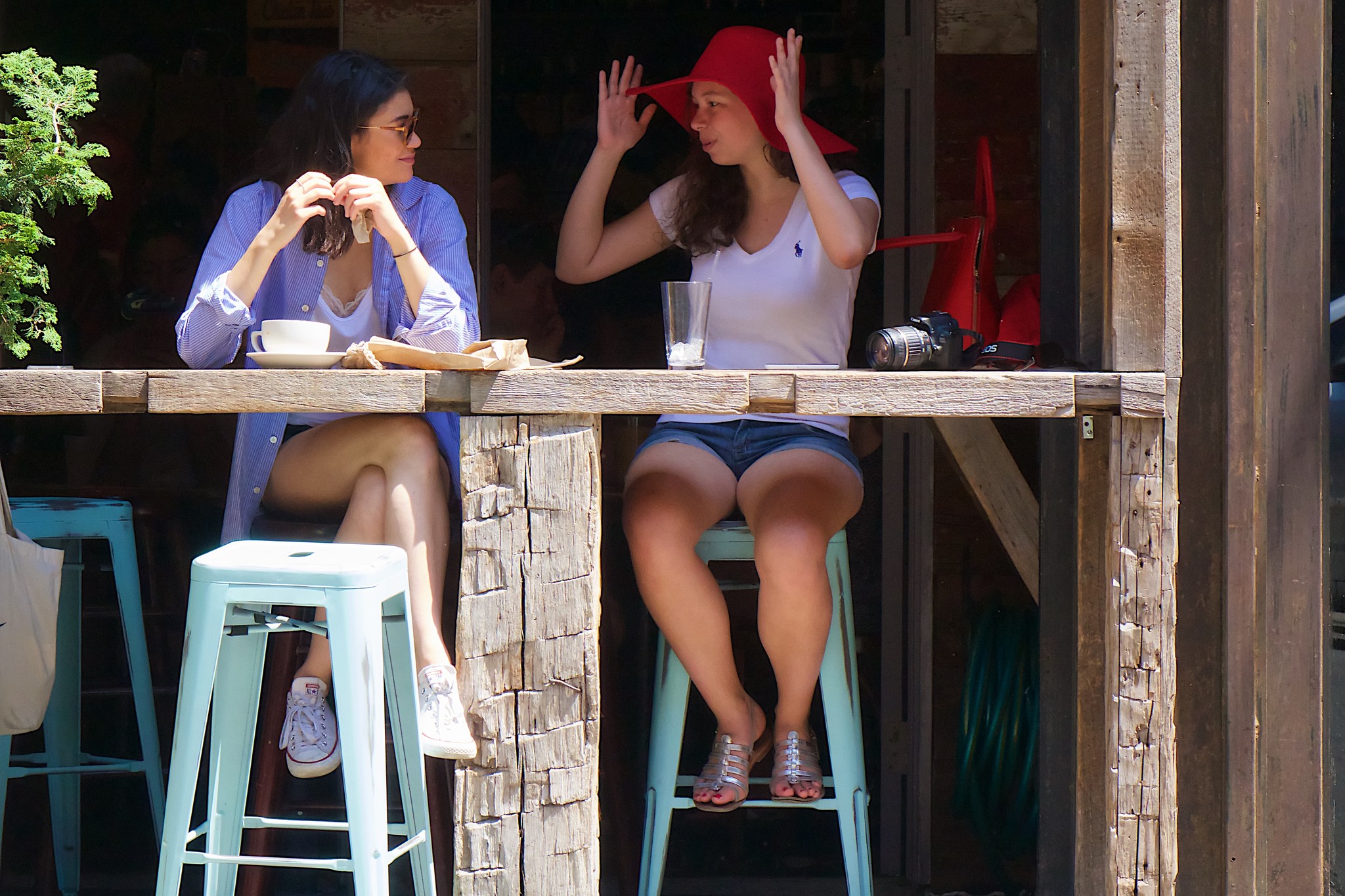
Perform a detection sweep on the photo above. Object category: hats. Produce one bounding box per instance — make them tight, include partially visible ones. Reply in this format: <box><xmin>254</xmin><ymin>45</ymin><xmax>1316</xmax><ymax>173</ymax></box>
<box><xmin>974</xmin><ymin>273</ymin><xmax>1041</xmax><ymax>370</ymax></box>
<box><xmin>625</xmin><ymin>26</ymin><xmax>858</xmax><ymax>154</ymax></box>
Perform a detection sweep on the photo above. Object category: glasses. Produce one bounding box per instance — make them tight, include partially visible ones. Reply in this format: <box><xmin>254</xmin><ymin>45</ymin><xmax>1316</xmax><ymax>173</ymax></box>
<box><xmin>358</xmin><ymin>106</ymin><xmax>421</xmax><ymax>147</ymax></box>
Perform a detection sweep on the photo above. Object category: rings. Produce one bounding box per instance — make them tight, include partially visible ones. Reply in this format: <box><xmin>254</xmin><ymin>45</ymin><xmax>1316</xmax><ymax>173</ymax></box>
<box><xmin>301</xmin><ymin>185</ymin><xmax>307</xmax><ymax>193</ymax></box>
<box><xmin>296</xmin><ymin>178</ymin><xmax>302</xmax><ymax>186</ymax></box>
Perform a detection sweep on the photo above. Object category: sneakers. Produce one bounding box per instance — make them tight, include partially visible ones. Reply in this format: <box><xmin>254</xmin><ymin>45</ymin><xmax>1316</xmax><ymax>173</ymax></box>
<box><xmin>417</xmin><ymin>664</ymin><xmax>477</xmax><ymax>759</ymax></box>
<box><xmin>277</xmin><ymin>675</ymin><xmax>342</xmax><ymax>779</ymax></box>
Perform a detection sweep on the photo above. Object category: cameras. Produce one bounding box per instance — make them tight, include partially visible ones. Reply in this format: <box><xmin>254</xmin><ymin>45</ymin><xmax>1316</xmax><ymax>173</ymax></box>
<box><xmin>868</xmin><ymin>309</ymin><xmax>964</xmax><ymax>371</ymax></box>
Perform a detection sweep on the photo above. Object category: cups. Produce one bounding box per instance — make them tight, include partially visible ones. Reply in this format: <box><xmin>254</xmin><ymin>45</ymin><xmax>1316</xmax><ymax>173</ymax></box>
<box><xmin>660</xmin><ymin>281</ymin><xmax>712</xmax><ymax>371</ymax></box>
<box><xmin>250</xmin><ymin>320</ymin><xmax>331</xmax><ymax>352</ymax></box>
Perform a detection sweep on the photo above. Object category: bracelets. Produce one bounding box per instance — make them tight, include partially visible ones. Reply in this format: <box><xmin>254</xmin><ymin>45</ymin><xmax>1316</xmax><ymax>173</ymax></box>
<box><xmin>392</xmin><ymin>247</ymin><xmax>417</xmax><ymax>259</ymax></box>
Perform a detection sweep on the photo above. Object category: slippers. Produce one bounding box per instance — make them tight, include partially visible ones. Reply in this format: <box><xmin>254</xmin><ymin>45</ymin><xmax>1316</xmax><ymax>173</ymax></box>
<box><xmin>768</xmin><ymin>720</ymin><xmax>827</xmax><ymax>805</ymax></box>
<box><xmin>693</xmin><ymin>727</ymin><xmax>774</xmax><ymax>813</ymax></box>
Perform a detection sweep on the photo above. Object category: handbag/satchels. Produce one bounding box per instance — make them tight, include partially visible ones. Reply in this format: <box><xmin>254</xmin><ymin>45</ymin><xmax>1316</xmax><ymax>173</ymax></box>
<box><xmin>0</xmin><ymin>457</ymin><xmax>65</xmax><ymax>738</ymax></box>
<box><xmin>871</xmin><ymin>134</ymin><xmax>999</xmax><ymax>360</ymax></box>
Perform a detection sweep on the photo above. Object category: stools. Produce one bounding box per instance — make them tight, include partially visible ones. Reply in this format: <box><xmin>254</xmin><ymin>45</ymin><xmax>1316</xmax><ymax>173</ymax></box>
<box><xmin>641</xmin><ymin>521</ymin><xmax>876</xmax><ymax>896</ymax></box>
<box><xmin>239</xmin><ymin>518</ymin><xmax>457</xmax><ymax>896</ymax></box>
<box><xmin>0</xmin><ymin>497</ymin><xmax>165</xmax><ymax>896</ymax></box>
<box><xmin>32</xmin><ymin>504</ymin><xmax>191</xmax><ymax>896</ymax></box>
<box><xmin>157</xmin><ymin>540</ymin><xmax>437</xmax><ymax>896</ymax></box>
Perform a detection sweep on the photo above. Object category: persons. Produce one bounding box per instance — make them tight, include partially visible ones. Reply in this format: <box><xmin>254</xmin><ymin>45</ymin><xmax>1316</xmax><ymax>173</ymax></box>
<box><xmin>556</xmin><ymin>26</ymin><xmax>884</xmax><ymax>814</ymax></box>
<box><xmin>174</xmin><ymin>48</ymin><xmax>482</xmax><ymax>777</ymax></box>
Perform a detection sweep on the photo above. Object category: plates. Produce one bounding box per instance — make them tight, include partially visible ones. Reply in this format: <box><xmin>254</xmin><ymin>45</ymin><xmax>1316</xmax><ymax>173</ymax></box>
<box><xmin>247</xmin><ymin>352</ymin><xmax>347</xmax><ymax>369</ymax></box>
